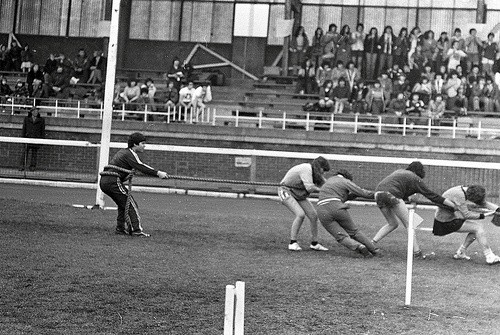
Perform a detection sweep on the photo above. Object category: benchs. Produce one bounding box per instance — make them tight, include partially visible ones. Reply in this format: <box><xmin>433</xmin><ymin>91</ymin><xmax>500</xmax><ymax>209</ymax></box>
<box><xmin>0</xmin><ymin>56</ymin><xmax>500</xmax><ymax>137</ymax></box>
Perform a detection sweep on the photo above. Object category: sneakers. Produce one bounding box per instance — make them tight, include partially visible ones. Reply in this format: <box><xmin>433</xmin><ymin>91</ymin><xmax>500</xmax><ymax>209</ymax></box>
<box><xmin>453</xmin><ymin>252</ymin><xmax>471</xmax><ymax>260</ymax></box>
<box><xmin>359</xmin><ymin>246</ymin><xmax>385</xmax><ymax>258</ymax></box>
<box><xmin>288</xmin><ymin>243</ymin><xmax>303</xmax><ymax>250</ymax></box>
<box><xmin>413</xmin><ymin>250</ymin><xmax>434</xmax><ymax>259</ymax></box>
<box><xmin>113</xmin><ymin>229</ymin><xmax>151</xmax><ymax>236</ymax></box>
<box><xmin>485</xmin><ymin>251</ymin><xmax>500</xmax><ymax>264</ymax></box>
<box><xmin>310</xmin><ymin>244</ymin><xmax>329</xmax><ymax>250</ymax></box>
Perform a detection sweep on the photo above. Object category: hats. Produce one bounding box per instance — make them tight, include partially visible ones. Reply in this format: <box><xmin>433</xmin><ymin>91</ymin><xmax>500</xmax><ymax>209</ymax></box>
<box><xmin>30</xmin><ymin>106</ymin><xmax>40</xmax><ymax>111</ymax></box>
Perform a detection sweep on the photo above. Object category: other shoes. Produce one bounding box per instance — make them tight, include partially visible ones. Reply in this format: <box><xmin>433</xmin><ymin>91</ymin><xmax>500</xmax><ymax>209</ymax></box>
<box><xmin>19</xmin><ymin>166</ymin><xmax>36</xmax><ymax>171</ymax></box>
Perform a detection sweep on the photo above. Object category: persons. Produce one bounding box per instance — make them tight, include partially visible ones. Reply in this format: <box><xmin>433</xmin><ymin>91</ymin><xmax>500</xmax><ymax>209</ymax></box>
<box><xmin>100</xmin><ymin>132</ymin><xmax>170</xmax><ymax>239</ymax></box>
<box><xmin>371</xmin><ymin>161</ymin><xmax>460</xmax><ymax>259</ymax></box>
<box><xmin>278</xmin><ymin>157</ymin><xmax>331</xmax><ymax>253</ymax></box>
<box><xmin>288</xmin><ymin>21</ymin><xmax>500</xmax><ymax>135</ymax></box>
<box><xmin>18</xmin><ymin>107</ymin><xmax>47</xmax><ymax>172</ymax></box>
<box><xmin>1</xmin><ymin>36</ymin><xmax>215</xmax><ymax>124</ymax></box>
<box><xmin>317</xmin><ymin>169</ymin><xmax>385</xmax><ymax>258</ymax></box>
<box><xmin>432</xmin><ymin>184</ymin><xmax>500</xmax><ymax>266</ymax></box>
